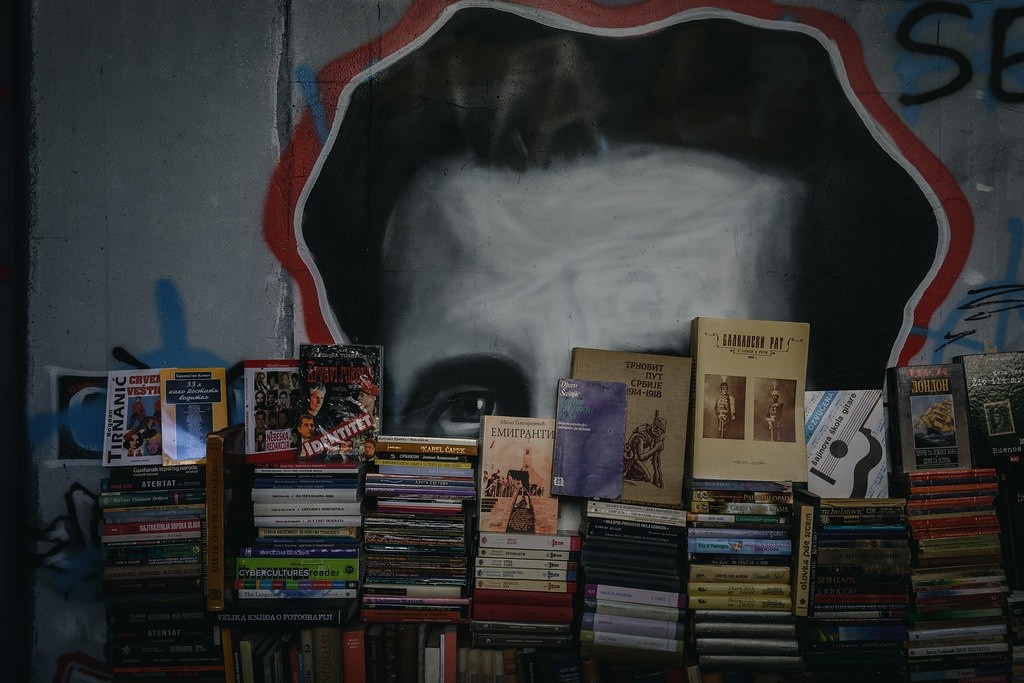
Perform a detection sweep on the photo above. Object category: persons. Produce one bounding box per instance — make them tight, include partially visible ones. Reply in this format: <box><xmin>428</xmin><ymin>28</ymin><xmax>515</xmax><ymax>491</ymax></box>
<box><xmin>299</xmin><ymin>377</ymin><xmax>379</xmax><ymax>462</ymax></box>
<box><xmin>767</xmin><ymin>391</ymin><xmax>784</xmax><ymax>442</ymax></box>
<box><xmin>299</xmin><ymin>6</ymin><xmax>940</xmax><ymax>434</ymax></box>
<box><xmin>123</xmin><ymin>430</ymin><xmax>143</xmax><ymax>457</ymax></box>
<box><xmin>714</xmin><ymin>382</ymin><xmax>736</xmax><ymax>438</ymax></box>
<box><xmin>144</xmin><ymin>432</ymin><xmax>162</xmax><ymax>455</ymax></box>
<box><xmin>253</xmin><ymin>371</ymin><xmax>300</xmax><ymax>452</ymax></box>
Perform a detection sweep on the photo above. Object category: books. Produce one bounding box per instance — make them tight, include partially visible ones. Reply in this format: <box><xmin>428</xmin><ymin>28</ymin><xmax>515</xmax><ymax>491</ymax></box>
<box><xmin>298</xmin><ymin>344</ymin><xmax>385</xmax><ymax>461</ymax></box>
<box><xmin>96</xmin><ymin>436</ymin><xmax>1024</xmax><ymax>683</ymax></box>
<box><xmin>686</xmin><ymin>317</ymin><xmax>1024</xmax><ymax>500</ymax></box>
<box><xmin>244</xmin><ymin>359</ymin><xmax>299</xmax><ymax>464</ymax></box>
<box><xmin>102</xmin><ymin>367</ymin><xmax>228</xmax><ymax>467</ymax></box>
<box><xmin>551</xmin><ymin>347</ymin><xmax>692</xmax><ymax>509</ymax></box>
<box><xmin>475</xmin><ymin>415</ymin><xmax>560</xmax><ymax>535</ymax></box>
